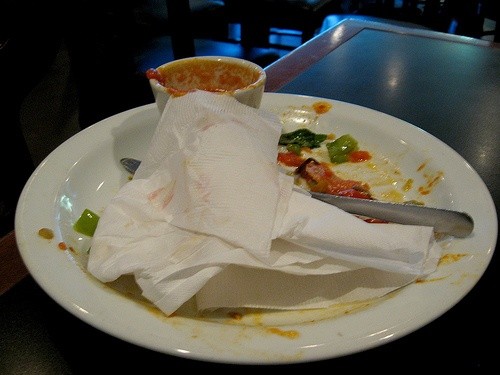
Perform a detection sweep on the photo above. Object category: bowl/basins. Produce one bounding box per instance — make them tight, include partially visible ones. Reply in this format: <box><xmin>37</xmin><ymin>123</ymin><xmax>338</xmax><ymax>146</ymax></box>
<box><xmin>151</xmin><ymin>55</ymin><xmax>267</xmax><ymax>123</ymax></box>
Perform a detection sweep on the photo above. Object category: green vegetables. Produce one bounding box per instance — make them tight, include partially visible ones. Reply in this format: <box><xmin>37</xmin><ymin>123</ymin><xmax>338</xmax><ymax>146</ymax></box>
<box><xmin>73</xmin><ymin>128</ymin><xmax>358</xmax><ymax>236</ymax></box>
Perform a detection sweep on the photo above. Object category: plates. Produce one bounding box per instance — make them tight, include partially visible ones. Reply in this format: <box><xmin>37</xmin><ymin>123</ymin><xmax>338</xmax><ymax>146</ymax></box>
<box><xmin>14</xmin><ymin>90</ymin><xmax>500</xmax><ymax>368</ymax></box>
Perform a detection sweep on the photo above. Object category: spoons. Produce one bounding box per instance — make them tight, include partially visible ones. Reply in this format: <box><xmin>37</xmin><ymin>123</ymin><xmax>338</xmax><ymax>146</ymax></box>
<box><xmin>121</xmin><ymin>158</ymin><xmax>476</xmax><ymax>237</ymax></box>
